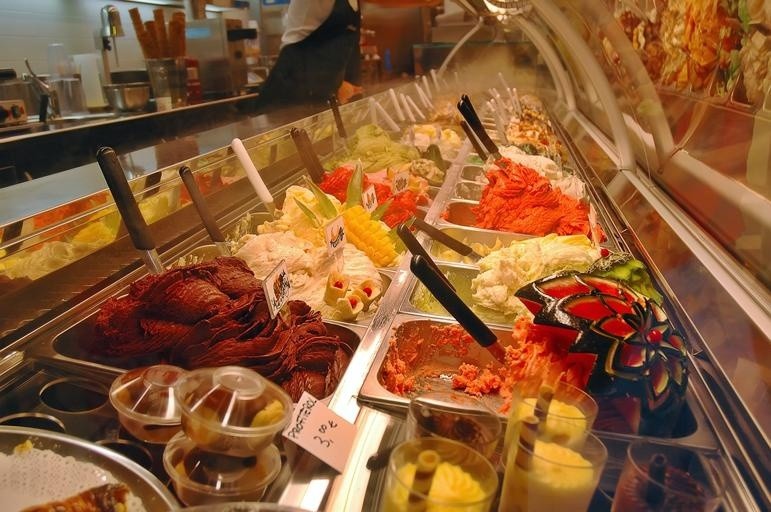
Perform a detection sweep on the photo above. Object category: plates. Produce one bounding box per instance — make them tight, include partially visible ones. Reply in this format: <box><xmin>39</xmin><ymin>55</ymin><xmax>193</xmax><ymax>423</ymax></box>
<box><xmin>0</xmin><ymin>423</ymin><xmax>181</xmax><ymax>511</ymax></box>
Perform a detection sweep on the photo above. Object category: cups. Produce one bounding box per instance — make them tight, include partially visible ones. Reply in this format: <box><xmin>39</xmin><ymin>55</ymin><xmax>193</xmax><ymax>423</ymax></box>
<box><xmin>46</xmin><ymin>43</ymin><xmax>76</xmax><ymax>81</ymax></box>
<box><xmin>497</xmin><ymin>376</ymin><xmax>599</xmax><ymax>480</ymax></box>
<box><xmin>373</xmin><ymin>437</ymin><xmax>500</xmax><ymax>511</ymax></box>
<box><xmin>498</xmin><ymin>416</ymin><xmax>609</xmax><ymax>509</ymax></box>
<box><xmin>610</xmin><ymin>438</ymin><xmax>725</xmax><ymax>512</ymax></box>
<box><xmin>404</xmin><ymin>389</ymin><xmax>502</xmax><ymax>458</ymax></box>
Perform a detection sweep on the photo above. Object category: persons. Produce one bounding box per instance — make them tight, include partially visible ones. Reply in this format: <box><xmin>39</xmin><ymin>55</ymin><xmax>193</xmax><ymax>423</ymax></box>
<box><xmin>249</xmin><ymin>0</ymin><xmax>364</xmax><ymax>116</ymax></box>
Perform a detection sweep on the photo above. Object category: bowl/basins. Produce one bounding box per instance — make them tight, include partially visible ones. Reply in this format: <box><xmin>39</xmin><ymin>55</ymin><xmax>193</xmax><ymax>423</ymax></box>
<box><xmin>101</xmin><ymin>80</ymin><xmax>150</xmax><ymax>114</ymax></box>
<box><xmin>108</xmin><ymin>364</ymin><xmax>295</xmax><ymax>508</ymax></box>
